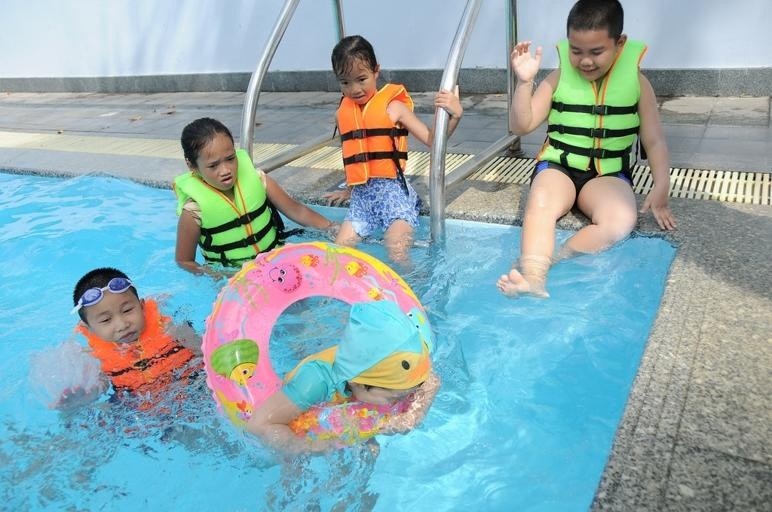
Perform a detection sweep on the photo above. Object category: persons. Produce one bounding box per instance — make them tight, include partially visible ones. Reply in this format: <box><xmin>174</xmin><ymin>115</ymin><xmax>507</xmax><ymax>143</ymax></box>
<box><xmin>51</xmin><ymin>267</ymin><xmax>205</xmax><ymax>412</ymax></box>
<box><xmin>174</xmin><ymin>117</ymin><xmax>342</xmax><ymax>283</ymax></box>
<box><xmin>246</xmin><ymin>296</ymin><xmax>441</xmax><ymax>456</ymax></box>
<box><xmin>496</xmin><ymin>1</ymin><xmax>679</xmax><ymax>301</ymax></box>
<box><xmin>321</xmin><ymin>35</ymin><xmax>463</xmax><ymax>274</ymax></box>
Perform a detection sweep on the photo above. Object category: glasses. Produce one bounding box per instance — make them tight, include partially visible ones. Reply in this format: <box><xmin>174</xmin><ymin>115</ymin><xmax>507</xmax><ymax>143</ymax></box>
<box><xmin>70</xmin><ymin>278</ymin><xmax>132</xmax><ymax>314</ymax></box>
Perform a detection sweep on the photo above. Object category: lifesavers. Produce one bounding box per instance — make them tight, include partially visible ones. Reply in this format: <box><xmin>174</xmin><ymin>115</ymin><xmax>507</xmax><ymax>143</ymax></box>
<box><xmin>201</xmin><ymin>242</ymin><xmax>441</xmax><ymax>453</ymax></box>
